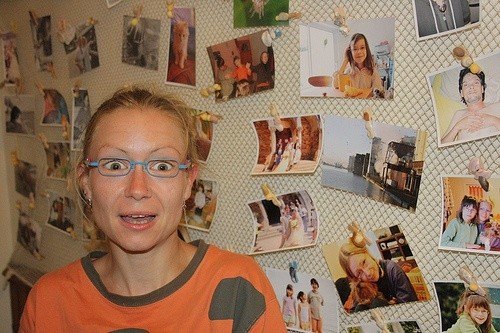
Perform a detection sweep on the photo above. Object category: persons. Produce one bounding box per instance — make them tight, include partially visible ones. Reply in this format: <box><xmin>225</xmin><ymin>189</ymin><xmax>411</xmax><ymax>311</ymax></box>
<box><xmin>17</xmin><ymin>86</ymin><xmax>289</xmax><ymax>333</ymax></box>
<box><xmin>5</xmin><ymin>40</ymin><xmax>19</xmax><ymax>83</ymax></box>
<box><xmin>279</xmin><ymin>201</ymin><xmax>308</xmax><ymax>249</ymax></box>
<box><xmin>441</xmin><ymin>290</ymin><xmax>497</xmax><ymax>333</ymax></box>
<box><xmin>53</xmin><ymin>146</ymin><xmax>61</xmax><ymax>170</ymax></box>
<box><xmin>194</xmin><ymin>184</ymin><xmax>206</xmax><ymax>217</ymax></box>
<box><xmin>442</xmin><ymin>68</ymin><xmax>500</xmax><ymax>143</ymax></box>
<box><xmin>73</xmin><ymin>35</ymin><xmax>94</xmax><ymax>75</ymax></box>
<box><xmin>6</xmin><ymin>105</ymin><xmax>27</xmax><ymax>134</ymax></box>
<box><xmin>336</xmin><ymin>33</ymin><xmax>383</xmax><ymax>101</ymax></box>
<box><xmin>50</xmin><ymin>202</ymin><xmax>74</xmax><ymax>233</ymax></box>
<box><xmin>441</xmin><ymin>196</ymin><xmax>500</xmax><ymax>250</ymax></box>
<box><xmin>263</xmin><ymin>138</ymin><xmax>301</xmax><ymax>172</ymax></box>
<box><xmin>213</xmin><ymin>51</ymin><xmax>273</xmax><ymax>101</ymax></box>
<box><xmin>339</xmin><ymin>243</ymin><xmax>419</xmax><ymax>313</ymax></box>
<box><xmin>281</xmin><ymin>278</ymin><xmax>323</xmax><ymax>333</ymax></box>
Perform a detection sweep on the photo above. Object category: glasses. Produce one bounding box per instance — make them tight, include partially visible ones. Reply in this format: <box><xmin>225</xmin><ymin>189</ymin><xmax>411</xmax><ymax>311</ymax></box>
<box><xmin>83</xmin><ymin>156</ymin><xmax>192</xmax><ymax>178</ymax></box>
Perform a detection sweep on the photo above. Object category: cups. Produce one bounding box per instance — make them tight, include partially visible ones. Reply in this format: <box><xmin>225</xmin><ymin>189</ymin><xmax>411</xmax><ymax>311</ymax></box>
<box><xmin>338</xmin><ymin>74</ymin><xmax>351</xmax><ymax>92</ymax></box>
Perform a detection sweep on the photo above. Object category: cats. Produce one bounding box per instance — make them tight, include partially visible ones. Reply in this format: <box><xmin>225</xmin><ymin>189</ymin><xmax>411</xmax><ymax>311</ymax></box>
<box><xmin>172</xmin><ymin>20</ymin><xmax>190</xmax><ymax>68</ymax></box>
